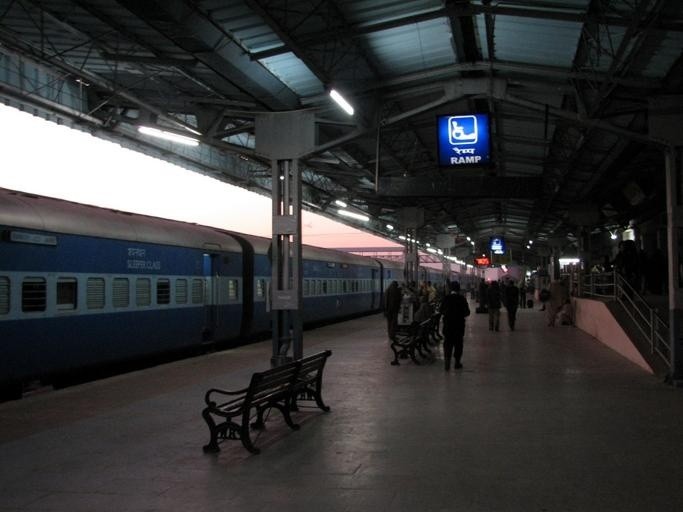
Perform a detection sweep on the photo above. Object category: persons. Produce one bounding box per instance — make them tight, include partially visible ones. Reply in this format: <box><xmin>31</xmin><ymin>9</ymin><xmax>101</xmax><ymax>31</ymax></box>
<box><xmin>594</xmin><ymin>240</ymin><xmax>667</xmax><ymax>300</ymax></box>
<box><xmin>385</xmin><ymin>278</ymin><xmax>574</xmax><ymax>370</ymax></box>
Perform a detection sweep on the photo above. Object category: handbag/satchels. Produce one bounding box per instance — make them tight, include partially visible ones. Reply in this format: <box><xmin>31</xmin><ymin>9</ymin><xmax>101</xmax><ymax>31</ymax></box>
<box><xmin>539</xmin><ymin>288</ymin><xmax>552</xmax><ymax>302</ymax></box>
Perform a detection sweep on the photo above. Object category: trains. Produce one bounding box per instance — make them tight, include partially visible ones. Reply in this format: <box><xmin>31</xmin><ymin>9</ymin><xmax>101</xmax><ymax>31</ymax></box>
<box><xmin>1</xmin><ymin>185</ymin><xmax>488</xmax><ymax>394</ymax></box>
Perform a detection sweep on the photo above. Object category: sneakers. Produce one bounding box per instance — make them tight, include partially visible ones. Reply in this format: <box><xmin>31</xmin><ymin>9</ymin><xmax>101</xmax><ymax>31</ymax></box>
<box><xmin>455</xmin><ymin>359</ymin><xmax>462</xmax><ymax>369</ymax></box>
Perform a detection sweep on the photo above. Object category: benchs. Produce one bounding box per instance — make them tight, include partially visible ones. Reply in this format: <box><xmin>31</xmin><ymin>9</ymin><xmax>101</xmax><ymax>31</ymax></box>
<box><xmin>391</xmin><ymin>313</ymin><xmax>443</xmax><ymax>365</ymax></box>
<box><xmin>202</xmin><ymin>350</ymin><xmax>333</xmax><ymax>456</ymax></box>
